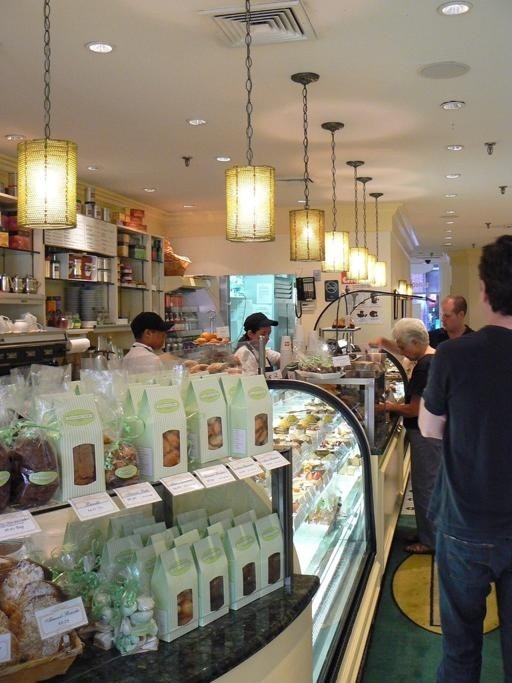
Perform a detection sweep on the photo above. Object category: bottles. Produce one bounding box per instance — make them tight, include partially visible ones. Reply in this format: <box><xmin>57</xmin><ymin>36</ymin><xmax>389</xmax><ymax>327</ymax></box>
<box><xmin>46</xmin><ymin>295</ymin><xmax>80</xmax><ymax>329</ymax></box>
<box><xmin>45</xmin><ymin>251</ymin><xmax>60</xmax><ymax>279</ymax></box>
<box><xmin>164</xmin><ymin>312</ymin><xmax>185</xmax><ymax>332</ymax></box>
<box><xmin>98</xmin><ymin>256</ymin><xmax>111</xmax><ymax>282</ymax></box>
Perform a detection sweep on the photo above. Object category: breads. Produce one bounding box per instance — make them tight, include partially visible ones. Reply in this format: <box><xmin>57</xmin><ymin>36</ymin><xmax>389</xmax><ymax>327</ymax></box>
<box><xmin>195</xmin><ymin>332</ymin><xmax>229</xmax><ymax>344</ymax></box>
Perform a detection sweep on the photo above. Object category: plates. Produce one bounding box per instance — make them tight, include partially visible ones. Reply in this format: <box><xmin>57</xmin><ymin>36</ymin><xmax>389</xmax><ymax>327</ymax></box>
<box><xmin>63</xmin><ymin>286</ymin><xmax>105</xmax><ymax>320</ymax></box>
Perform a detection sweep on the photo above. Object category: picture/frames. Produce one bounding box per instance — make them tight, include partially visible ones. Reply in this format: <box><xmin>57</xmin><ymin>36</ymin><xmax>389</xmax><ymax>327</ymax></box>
<box><xmin>401</xmin><ymin>298</ymin><xmax>406</xmax><ymax>319</ymax></box>
<box><xmin>393</xmin><ymin>288</ymin><xmax>399</xmax><ymax>319</ymax></box>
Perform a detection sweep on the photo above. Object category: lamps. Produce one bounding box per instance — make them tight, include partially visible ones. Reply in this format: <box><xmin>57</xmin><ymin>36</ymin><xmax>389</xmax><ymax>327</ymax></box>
<box><xmin>11</xmin><ymin>1</ymin><xmax>82</xmax><ymax>232</ymax></box>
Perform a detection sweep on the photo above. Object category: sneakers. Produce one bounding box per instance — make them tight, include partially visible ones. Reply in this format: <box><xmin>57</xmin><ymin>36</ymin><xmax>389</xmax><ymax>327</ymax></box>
<box><xmin>402</xmin><ymin>534</ymin><xmax>420</xmax><ymax>543</ymax></box>
<box><xmin>402</xmin><ymin>543</ymin><xmax>437</xmax><ymax>555</ymax></box>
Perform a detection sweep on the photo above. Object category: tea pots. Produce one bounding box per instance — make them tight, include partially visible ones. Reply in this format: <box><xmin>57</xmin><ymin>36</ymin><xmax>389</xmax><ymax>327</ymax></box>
<box><xmin>2</xmin><ymin>274</ymin><xmax>41</xmax><ymax>296</ymax></box>
<box><xmin>0</xmin><ymin>311</ymin><xmax>41</xmax><ymax>333</ymax></box>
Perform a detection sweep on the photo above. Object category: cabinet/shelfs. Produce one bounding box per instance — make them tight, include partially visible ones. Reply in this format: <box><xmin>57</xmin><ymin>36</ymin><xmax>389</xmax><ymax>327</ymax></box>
<box><xmin>1</xmin><ymin>190</ymin><xmax>212</xmax><ymax>358</ymax></box>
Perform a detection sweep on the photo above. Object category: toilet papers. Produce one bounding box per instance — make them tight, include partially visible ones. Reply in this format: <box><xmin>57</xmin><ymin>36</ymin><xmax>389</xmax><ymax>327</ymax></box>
<box><xmin>65</xmin><ymin>338</ymin><xmax>91</xmax><ymax>354</ymax></box>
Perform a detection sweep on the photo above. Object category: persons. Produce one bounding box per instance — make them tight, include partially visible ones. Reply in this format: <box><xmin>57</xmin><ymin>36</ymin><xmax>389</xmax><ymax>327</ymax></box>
<box><xmin>124</xmin><ymin>312</ymin><xmax>175</xmax><ymax>371</ymax></box>
<box><xmin>367</xmin><ymin>293</ymin><xmax>476</xmax><ymax>346</ymax></box>
<box><xmin>233</xmin><ymin>312</ymin><xmax>282</xmax><ymax>373</ymax></box>
<box><xmin>415</xmin><ymin>233</ymin><xmax>512</xmax><ymax>682</ymax></box>
<box><xmin>370</xmin><ymin>316</ymin><xmax>440</xmax><ymax>555</ymax></box>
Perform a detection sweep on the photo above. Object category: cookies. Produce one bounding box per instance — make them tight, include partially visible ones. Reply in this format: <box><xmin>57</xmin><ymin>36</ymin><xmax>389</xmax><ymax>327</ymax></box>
<box><xmin>73</xmin><ymin>445</ymin><xmax>96</xmax><ymax>485</ymax></box>
<box><xmin>163</xmin><ymin>430</ymin><xmax>180</xmax><ymax>467</ymax></box>
<box><xmin>207</xmin><ymin>417</ymin><xmax>223</xmax><ymax>450</ymax></box>
<box><xmin>256</xmin><ymin>416</ymin><xmax>268</xmax><ymax>445</ymax></box>
<box><xmin>178</xmin><ymin>592</ymin><xmax>193</xmax><ymax>625</ymax></box>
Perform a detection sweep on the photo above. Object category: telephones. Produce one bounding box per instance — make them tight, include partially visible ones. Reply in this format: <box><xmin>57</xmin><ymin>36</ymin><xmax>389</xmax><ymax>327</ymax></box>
<box><xmin>296</xmin><ymin>277</ymin><xmax>316</xmax><ymax>300</ymax></box>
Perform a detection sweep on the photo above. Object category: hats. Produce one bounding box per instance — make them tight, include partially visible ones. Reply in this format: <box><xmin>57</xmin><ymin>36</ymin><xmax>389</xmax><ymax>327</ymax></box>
<box><xmin>243</xmin><ymin>312</ymin><xmax>280</xmax><ymax>332</ymax></box>
<box><xmin>129</xmin><ymin>310</ymin><xmax>176</xmax><ymax>336</ymax></box>
